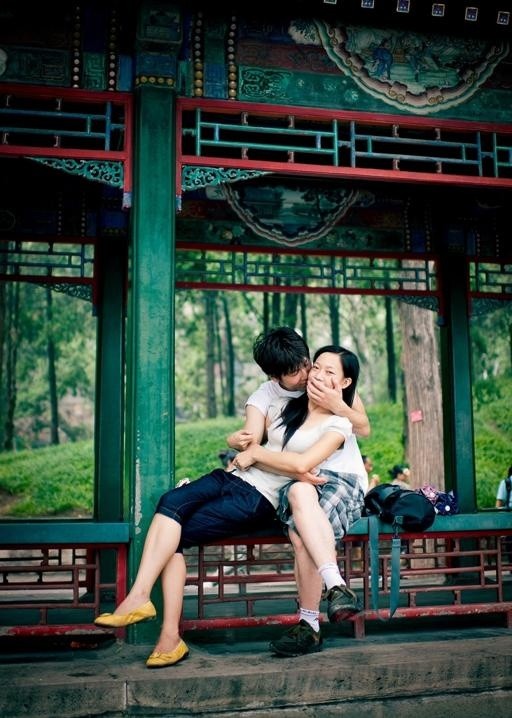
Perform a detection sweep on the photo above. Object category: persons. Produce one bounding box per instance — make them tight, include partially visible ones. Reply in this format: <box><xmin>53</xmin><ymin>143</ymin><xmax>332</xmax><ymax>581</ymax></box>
<box><xmin>96</xmin><ymin>346</ymin><xmax>361</xmax><ymax>668</ymax></box>
<box><xmin>496</xmin><ymin>466</ymin><xmax>512</xmax><ymax>574</ymax></box>
<box><xmin>218</xmin><ymin>449</ymin><xmax>239</xmax><ymax>472</ymax></box>
<box><xmin>363</xmin><ymin>456</ymin><xmax>411</xmax><ymax>493</ymax></box>
<box><xmin>227</xmin><ymin>327</ymin><xmax>368</xmax><ymax>657</ymax></box>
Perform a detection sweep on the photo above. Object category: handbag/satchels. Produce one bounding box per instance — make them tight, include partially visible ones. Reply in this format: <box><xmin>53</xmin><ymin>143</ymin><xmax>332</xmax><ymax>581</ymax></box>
<box><xmin>364</xmin><ymin>484</ymin><xmax>435</xmax><ymax>532</ymax></box>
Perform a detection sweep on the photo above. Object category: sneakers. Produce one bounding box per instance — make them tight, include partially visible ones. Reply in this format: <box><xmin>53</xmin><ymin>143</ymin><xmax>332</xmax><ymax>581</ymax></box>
<box><xmin>270</xmin><ymin>619</ymin><xmax>323</xmax><ymax>657</ymax></box>
<box><xmin>323</xmin><ymin>585</ymin><xmax>364</xmax><ymax>623</ymax></box>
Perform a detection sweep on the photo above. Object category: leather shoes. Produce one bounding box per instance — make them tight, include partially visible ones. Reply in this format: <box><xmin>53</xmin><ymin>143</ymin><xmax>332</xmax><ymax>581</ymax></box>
<box><xmin>94</xmin><ymin>602</ymin><xmax>156</xmax><ymax>627</ymax></box>
<box><xmin>146</xmin><ymin>638</ymin><xmax>189</xmax><ymax>667</ymax></box>
